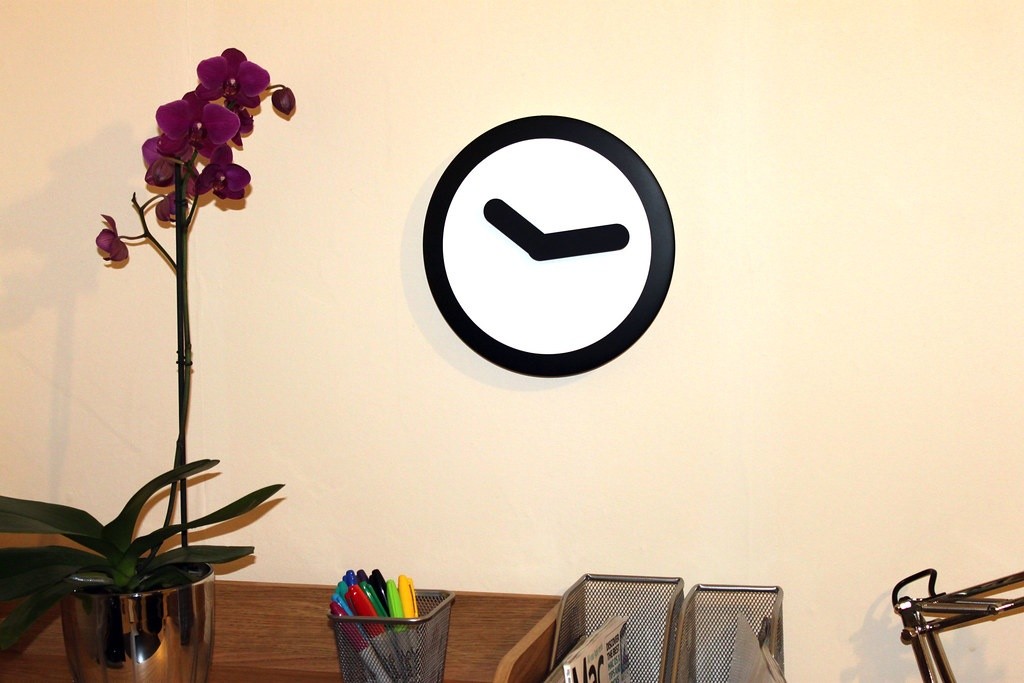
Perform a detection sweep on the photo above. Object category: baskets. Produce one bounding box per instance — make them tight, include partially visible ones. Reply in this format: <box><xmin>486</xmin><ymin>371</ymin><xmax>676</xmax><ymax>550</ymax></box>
<box><xmin>327</xmin><ymin>589</ymin><xmax>455</xmax><ymax>683</ymax></box>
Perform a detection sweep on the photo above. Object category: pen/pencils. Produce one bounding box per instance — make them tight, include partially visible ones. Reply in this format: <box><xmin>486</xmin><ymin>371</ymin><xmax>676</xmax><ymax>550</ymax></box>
<box><xmin>330</xmin><ymin>568</ymin><xmax>422</xmax><ymax>683</ymax></box>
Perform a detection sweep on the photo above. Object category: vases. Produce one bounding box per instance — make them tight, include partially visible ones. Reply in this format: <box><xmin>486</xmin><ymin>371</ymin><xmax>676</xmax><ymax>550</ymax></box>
<box><xmin>60</xmin><ymin>564</ymin><xmax>215</xmax><ymax>683</ymax></box>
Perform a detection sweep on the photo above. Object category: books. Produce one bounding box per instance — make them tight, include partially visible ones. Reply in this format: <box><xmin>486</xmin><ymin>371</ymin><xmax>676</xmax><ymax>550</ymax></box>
<box><xmin>746</xmin><ymin>645</ymin><xmax>787</xmax><ymax>683</ymax></box>
<box><xmin>544</xmin><ymin>616</ymin><xmax>632</xmax><ymax>683</ymax></box>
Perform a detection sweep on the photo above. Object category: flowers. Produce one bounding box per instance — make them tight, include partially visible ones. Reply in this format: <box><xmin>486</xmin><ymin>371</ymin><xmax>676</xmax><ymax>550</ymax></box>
<box><xmin>1</xmin><ymin>47</ymin><xmax>295</xmax><ymax>648</ymax></box>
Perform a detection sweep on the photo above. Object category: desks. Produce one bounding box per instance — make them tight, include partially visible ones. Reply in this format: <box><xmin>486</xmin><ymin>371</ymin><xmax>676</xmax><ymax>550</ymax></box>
<box><xmin>0</xmin><ymin>579</ymin><xmax>563</xmax><ymax>683</ymax></box>
